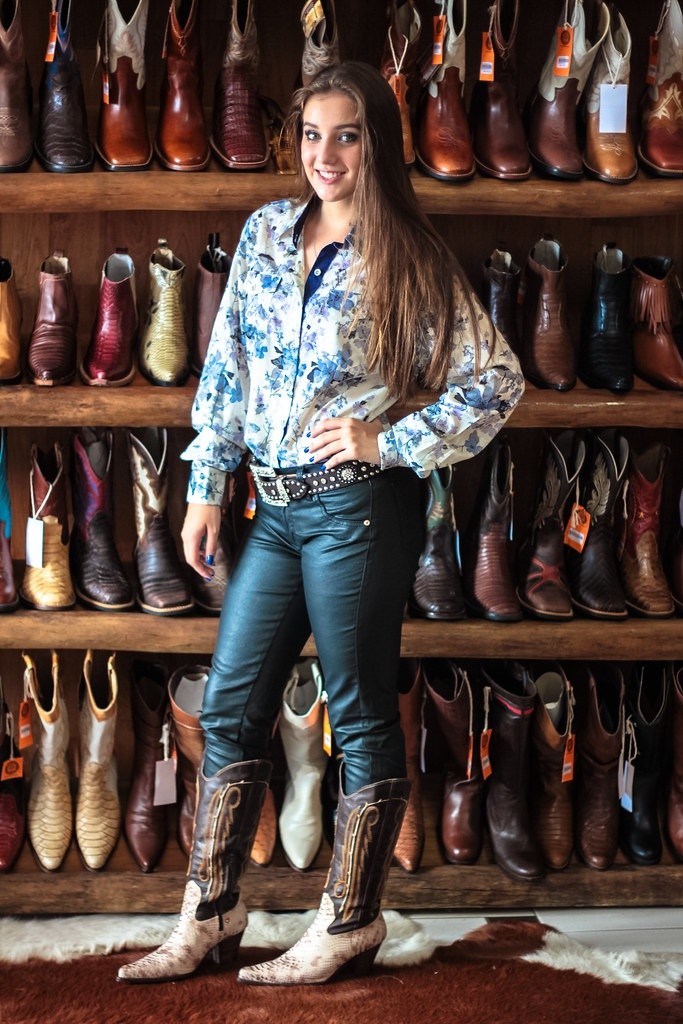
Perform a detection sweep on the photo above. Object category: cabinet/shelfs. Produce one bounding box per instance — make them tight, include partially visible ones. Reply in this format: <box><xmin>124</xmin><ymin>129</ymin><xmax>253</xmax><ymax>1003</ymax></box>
<box><xmin>0</xmin><ymin>152</ymin><xmax>683</xmax><ymax>916</ymax></box>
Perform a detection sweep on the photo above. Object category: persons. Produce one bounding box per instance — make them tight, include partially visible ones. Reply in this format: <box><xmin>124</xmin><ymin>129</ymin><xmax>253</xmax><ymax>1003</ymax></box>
<box><xmin>115</xmin><ymin>59</ymin><xmax>531</xmax><ymax>992</ymax></box>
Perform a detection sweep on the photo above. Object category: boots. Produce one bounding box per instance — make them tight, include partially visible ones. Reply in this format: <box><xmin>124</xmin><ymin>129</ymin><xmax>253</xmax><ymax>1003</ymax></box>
<box><xmin>236</xmin><ymin>761</ymin><xmax>412</xmax><ymax>987</ymax></box>
<box><xmin>0</xmin><ymin>233</ymin><xmax>231</xmax><ymax>387</ymax></box>
<box><xmin>0</xmin><ymin>0</ymin><xmax>683</xmax><ymax>184</ymax></box>
<box><xmin>412</xmin><ymin>435</ymin><xmax>683</xmax><ymax>622</ymax></box>
<box><xmin>0</xmin><ymin>648</ymin><xmax>683</xmax><ymax>879</ymax></box>
<box><xmin>116</xmin><ymin>746</ymin><xmax>270</xmax><ymax>984</ymax></box>
<box><xmin>1</xmin><ymin>427</ymin><xmax>231</xmax><ymax>615</ymax></box>
<box><xmin>481</xmin><ymin>239</ymin><xmax>683</xmax><ymax>395</ymax></box>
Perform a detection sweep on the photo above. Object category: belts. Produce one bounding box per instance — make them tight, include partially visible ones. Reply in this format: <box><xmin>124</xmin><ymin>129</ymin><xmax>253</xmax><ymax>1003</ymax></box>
<box><xmin>247</xmin><ymin>455</ymin><xmax>381</xmax><ymax>508</ymax></box>
